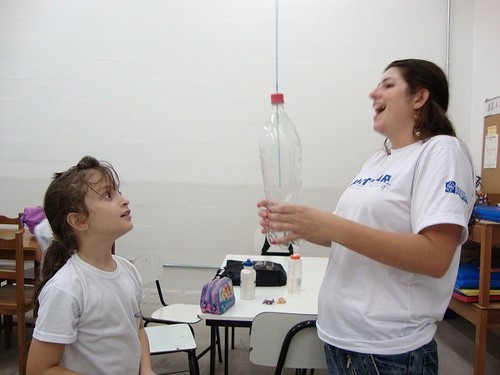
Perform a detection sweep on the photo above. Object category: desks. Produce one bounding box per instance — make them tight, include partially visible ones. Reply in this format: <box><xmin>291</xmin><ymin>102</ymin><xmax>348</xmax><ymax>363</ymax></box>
<box><xmin>0</xmin><ymin>228</ymin><xmax>41</xmax><ymax>319</ymax></box>
<box><xmin>197</xmin><ymin>254</ymin><xmax>329</xmax><ymax>375</ymax></box>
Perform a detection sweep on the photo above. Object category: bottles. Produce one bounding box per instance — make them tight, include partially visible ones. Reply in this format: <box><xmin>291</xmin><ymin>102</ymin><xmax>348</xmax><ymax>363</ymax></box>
<box><xmin>258</xmin><ymin>93</ymin><xmax>304</xmax><ymax>249</ymax></box>
<box><xmin>240</xmin><ymin>259</ymin><xmax>256</xmax><ymax>301</ymax></box>
<box><xmin>287</xmin><ymin>254</ymin><xmax>302</xmax><ymax>292</ymax></box>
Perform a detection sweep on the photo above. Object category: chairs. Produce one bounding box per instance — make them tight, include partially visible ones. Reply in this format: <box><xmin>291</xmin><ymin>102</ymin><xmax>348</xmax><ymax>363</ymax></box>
<box><xmin>0</xmin><ymin>213</ymin><xmax>37</xmax><ymax>375</ymax></box>
<box><xmin>133</xmin><ymin>252</ymin><xmax>223</xmax><ymax>375</ymax></box>
<box><xmin>249</xmin><ymin>312</ymin><xmax>328</xmax><ymax>375</ymax></box>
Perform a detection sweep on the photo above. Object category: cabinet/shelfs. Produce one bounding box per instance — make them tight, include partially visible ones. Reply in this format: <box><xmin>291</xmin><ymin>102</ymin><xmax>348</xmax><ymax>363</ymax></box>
<box><xmin>447</xmin><ymin>218</ymin><xmax>500</xmax><ymax>375</ymax></box>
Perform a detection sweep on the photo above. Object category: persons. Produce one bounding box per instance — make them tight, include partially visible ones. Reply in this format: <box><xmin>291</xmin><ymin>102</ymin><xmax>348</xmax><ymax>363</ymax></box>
<box><xmin>256</xmin><ymin>57</ymin><xmax>477</xmax><ymax>375</ymax></box>
<box><xmin>24</xmin><ymin>156</ymin><xmax>159</xmax><ymax>375</ymax></box>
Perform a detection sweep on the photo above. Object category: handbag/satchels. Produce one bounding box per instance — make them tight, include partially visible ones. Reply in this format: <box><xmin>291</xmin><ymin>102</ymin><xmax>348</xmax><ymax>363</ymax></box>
<box><xmin>212</xmin><ymin>259</ymin><xmax>287</xmax><ymax>287</ymax></box>
<box><xmin>200</xmin><ymin>277</ymin><xmax>235</xmax><ymax>315</ymax></box>
